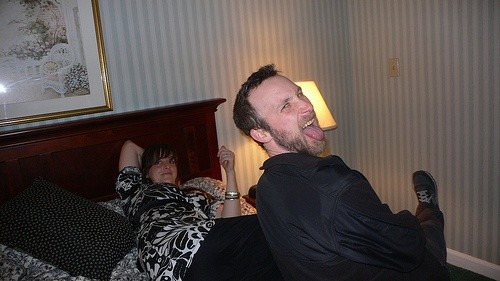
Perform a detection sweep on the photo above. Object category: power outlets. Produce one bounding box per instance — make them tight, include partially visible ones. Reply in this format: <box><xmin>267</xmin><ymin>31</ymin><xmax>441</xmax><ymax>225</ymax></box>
<box><xmin>388</xmin><ymin>59</ymin><xmax>399</xmax><ymax>77</ymax></box>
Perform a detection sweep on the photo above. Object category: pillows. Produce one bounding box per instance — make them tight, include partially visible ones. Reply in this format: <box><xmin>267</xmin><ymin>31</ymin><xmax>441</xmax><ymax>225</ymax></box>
<box><xmin>0</xmin><ymin>177</ymin><xmax>140</xmax><ymax>281</ymax></box>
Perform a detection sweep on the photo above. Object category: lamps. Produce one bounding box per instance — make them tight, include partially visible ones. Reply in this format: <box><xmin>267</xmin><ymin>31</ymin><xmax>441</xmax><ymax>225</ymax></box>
<box><xmin>293</xmin><ymin>81</ymin><xmax>337</xmax><ymax>131</ymax></box>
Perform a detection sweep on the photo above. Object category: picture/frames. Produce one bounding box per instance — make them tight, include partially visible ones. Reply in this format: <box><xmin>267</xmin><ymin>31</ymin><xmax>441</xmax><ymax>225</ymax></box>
<box><xmin>0</xmin><ymin>0</ymin><xmax>114</xmax><ymax>125</ymax></box>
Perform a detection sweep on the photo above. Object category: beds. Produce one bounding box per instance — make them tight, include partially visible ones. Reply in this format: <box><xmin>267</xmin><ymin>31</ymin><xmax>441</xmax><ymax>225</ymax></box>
<box><xmin>0</xmin><ymin>98</ymin><xmax>500</xmax><ymax>281</ymax></box>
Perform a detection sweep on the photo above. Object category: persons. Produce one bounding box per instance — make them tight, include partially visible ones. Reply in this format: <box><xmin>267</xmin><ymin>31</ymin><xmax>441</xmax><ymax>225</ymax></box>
<box><xmin>233</xmin><ymin>62</ymin><xmax>447</xmax><ymax>280</ymax></box>
<box><xmin>116</xmin><ymin>138</ymin><xmax>283</xmax><ymax>280</ymax></box>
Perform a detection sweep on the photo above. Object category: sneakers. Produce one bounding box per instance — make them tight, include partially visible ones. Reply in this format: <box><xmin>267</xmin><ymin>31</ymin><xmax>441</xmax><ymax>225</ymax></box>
<box><xmin>413</xmin><ymin>170</ymin><xmax>437</xmax><ymax>207</ymax></box>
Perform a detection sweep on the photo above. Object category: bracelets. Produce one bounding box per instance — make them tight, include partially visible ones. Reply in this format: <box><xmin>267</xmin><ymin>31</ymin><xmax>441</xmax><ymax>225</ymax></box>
<box><xmin>225</xmin><ymin>191</ymin><xmax>240</xmax><ymax>199</ymax></box>
